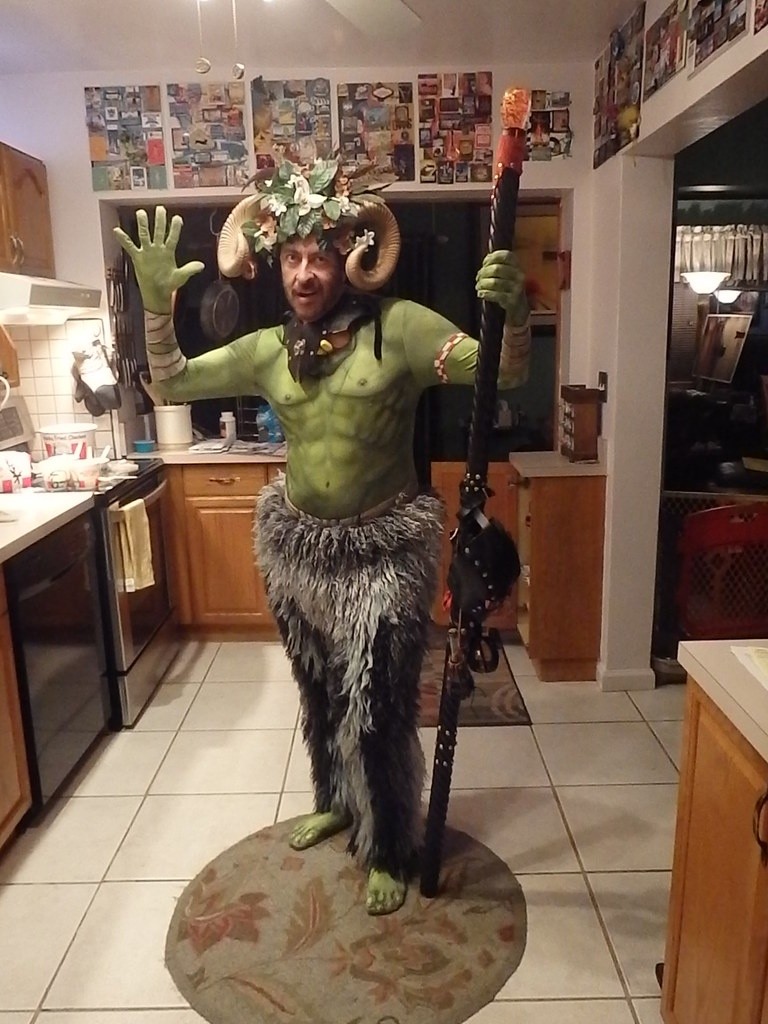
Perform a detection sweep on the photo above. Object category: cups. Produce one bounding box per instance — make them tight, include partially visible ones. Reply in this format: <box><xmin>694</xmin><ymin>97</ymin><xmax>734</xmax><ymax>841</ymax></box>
<box><xmin>40</xmin><ymin>455</ymin><xmax>110</xmax><ymax>493</ymax></box>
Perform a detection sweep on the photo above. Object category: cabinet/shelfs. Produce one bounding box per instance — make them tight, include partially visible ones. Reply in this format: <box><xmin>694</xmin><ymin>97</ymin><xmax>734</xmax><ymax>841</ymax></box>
<box><xmin>0</xmin><ymin>141</ymin><xmax>57</xmax><ymax>280</ymax></box>
<box><xmin>511</xmin><ymin>451</ymin><xmax>607</xmax><ymax>683</ymax></box>
<box><xmin>658</xmin><ymin>638</ymin><xmax>768</xmax><ymax>1024</ymax></box>
<box><xmin>129</xmin><ymin>441</ymin><xmax>288</xmax><ymax>643</ymax></box>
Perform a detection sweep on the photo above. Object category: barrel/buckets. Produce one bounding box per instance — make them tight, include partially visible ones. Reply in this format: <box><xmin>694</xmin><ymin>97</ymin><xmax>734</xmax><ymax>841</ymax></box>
<box><xmin>39</xmin><ymin>423</ymin><xmax>98</xmax><ymax>459</ymax></box>
<box><xmin>154</xmin><ymin>405</ymin><xmax>193</xmax><ymax>452</ymax></box>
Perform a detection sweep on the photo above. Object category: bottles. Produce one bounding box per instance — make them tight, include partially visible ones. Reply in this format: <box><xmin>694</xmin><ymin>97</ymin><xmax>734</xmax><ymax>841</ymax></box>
<box><xmin>220</xmin><ymin>412</ymin><xmax>236</xmax><ymax>439</ymax></box>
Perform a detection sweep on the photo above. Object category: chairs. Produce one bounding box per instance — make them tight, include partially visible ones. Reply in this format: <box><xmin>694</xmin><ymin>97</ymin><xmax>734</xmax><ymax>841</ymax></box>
<box><xmin>673</xmin><ymin>502</ymin><xmax>768</xmax><ymax>640</ymax></box>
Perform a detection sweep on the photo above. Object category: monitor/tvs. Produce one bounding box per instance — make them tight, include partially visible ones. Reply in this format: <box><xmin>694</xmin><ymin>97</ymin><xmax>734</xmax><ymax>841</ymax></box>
<box><xmin>692</xmin><ymin>315</ymin><xmax>753</xmax><ymax>384</ymax></box>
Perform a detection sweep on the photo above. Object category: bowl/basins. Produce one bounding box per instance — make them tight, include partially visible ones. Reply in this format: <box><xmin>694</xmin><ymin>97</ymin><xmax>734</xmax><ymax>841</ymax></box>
<box><xmin>133</xmin><ymin>440</ymin><xmax>155</xmax><ymax>452</ymax></box>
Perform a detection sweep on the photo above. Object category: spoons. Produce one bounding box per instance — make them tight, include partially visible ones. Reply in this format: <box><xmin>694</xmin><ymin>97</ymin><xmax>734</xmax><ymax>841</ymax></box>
<box><xmin>98</xmin><ymin>476</ymin><xmax>137</xmax><ymax>481</ymax></box>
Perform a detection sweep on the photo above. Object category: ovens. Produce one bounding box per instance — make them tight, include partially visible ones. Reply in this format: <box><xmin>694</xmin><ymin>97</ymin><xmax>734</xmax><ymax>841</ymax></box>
<box><xmin>95</xmin><ymin>473</ymin><xmax>183</xmax><ymax>727</ymax></box>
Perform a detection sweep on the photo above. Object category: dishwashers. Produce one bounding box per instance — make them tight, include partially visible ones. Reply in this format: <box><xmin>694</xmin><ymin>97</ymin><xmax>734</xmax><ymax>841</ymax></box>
<box><xmin>3</xmin><ymin>508</ymin><xmax>120</xmax><ymax>815</ymax></box>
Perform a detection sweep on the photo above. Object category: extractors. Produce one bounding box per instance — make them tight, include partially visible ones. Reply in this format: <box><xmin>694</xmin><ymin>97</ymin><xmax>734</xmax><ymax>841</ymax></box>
<box><xmin>0</xmin><ymin>273</ymin><xmax>102</xmax><ymax>326</ymax></box>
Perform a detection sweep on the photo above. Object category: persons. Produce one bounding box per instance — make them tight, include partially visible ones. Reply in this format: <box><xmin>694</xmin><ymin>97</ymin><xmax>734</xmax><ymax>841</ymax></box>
<box><xmin>113</xmin><ymin>142</ymin><xmax>533</xmax><ymax>915</ymax></box>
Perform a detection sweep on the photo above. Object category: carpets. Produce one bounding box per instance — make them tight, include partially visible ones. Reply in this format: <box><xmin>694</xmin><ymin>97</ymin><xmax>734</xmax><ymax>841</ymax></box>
<box><xmin>416</xmin><ymin>627</ymin><xmax>534</xmax><ymax>727</ymax></box>
<box><xmin>163</xmin><ymin>812</ymin><xmax>528</xmax><ymax>1024</ymax></box>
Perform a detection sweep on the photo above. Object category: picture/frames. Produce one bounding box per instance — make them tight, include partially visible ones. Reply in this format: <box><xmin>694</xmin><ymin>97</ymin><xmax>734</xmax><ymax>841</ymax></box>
<box><xmin>691</xmin><ymin>314</ymin><xmax>753</xmax><ymax>384</ymax></box>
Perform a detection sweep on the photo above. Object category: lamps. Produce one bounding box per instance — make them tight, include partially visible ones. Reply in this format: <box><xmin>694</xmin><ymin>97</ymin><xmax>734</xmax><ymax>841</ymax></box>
<box><xmin>713</xmin><ymin>290</ymin><xmax>744</xmax><ymax>304</ymax></box>
<box><xmin>680</xmin><ymin>271</ymin><xmax>732</xmax><ymax>295</ymax></box>
